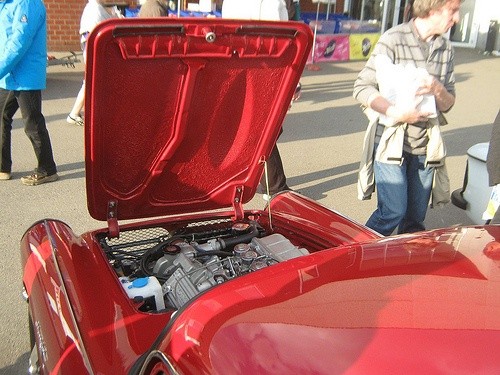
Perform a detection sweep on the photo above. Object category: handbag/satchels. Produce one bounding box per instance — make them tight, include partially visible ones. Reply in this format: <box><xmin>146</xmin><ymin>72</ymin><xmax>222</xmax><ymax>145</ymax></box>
<box><xmin>373</xmin><ymin>55</ymin><xmax>438</xmax><ymax>127</ymax></box>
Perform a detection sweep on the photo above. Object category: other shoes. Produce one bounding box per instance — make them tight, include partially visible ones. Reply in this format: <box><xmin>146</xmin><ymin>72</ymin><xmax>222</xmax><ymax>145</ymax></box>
<box><xmin>67</xmin><ymin>115</ymin><xmax>85</xmax><ymax>126</ymax></box>
<box><xmin>0</xmin><ymin>172</ymin><xmax>12</xmax><ymax>180</ymax></box>
<box><xmin>21</xmin><ymin>172</ymin><xmax>59</xmax><ymax>186</ymax></box>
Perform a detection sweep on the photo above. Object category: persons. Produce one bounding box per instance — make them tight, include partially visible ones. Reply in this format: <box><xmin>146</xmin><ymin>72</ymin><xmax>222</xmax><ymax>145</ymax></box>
<box><xmin>138</xmin><ymin>0</ymin><xmax>169</xmax><ymax>17</ymax></box>
<box><xmin>66</xmin><ymin>0</ymin><xmax>120</xmax><ymax>127</ymax></box>
<box><xmin>404</xmin><ymin>0</ymin><xmax>415</xmax><ymax>23</ymax></box>
<box><xmin>0</xmin><ymin>0</ymin><xmax>59</xmax><ymax>186</ymax></box>
<box><xmin>483</xmin><ymin>21</ymin><xmax>498</xmax><ymax>55</ymax></box>
<box><xmin>223</xmin><ymin>0</ymin><xmax>302</xmax><ymax>201</ymax></box>
<box><xmin>353</xmin><ymin>0</ymin><xmax>463</xmax><ymax>237</ymax></box>
<box><xmin>486</xmin><ymin>109</ymin><xmax>500</xmax><ymax>224</ymax></box>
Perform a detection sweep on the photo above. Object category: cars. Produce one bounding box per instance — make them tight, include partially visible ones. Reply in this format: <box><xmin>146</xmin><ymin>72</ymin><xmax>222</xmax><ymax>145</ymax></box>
<box><xmin>19</xmin><ymin>15</ymin><xmax>500</xmax><ymax>375</ymax></box>
